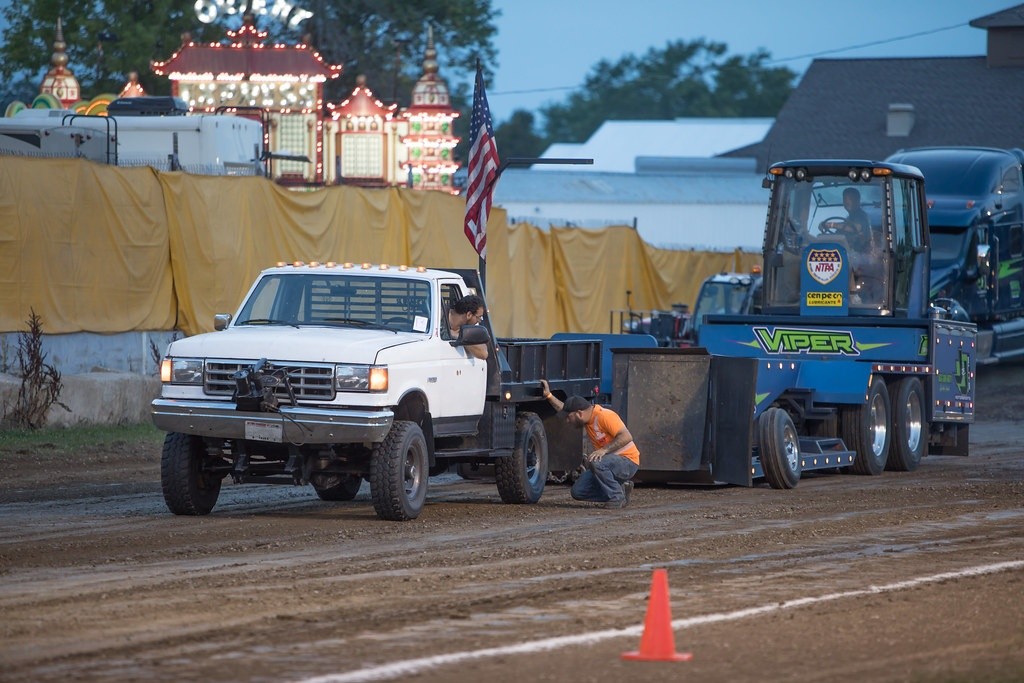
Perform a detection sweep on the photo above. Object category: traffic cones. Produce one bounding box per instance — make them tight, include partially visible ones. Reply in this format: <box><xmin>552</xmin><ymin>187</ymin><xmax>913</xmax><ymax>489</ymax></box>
<box><xmin>619</xmin><ymin>569</ymin><xmax>694</xmax><ymax>662</ymax></box>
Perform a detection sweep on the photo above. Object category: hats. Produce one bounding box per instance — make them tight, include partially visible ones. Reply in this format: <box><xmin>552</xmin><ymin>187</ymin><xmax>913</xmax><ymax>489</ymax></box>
<box><xmin>559</xmin><ymin>395</ymin><xmax>587</xmax><ymax>420</ymax></box>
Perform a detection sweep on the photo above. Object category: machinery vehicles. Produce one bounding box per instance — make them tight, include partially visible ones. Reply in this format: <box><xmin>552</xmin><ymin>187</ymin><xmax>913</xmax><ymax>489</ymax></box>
<box><xmin>546</xmin><ymin>157</ymin><xmax>981</xmax><ymax>489</ymax></box>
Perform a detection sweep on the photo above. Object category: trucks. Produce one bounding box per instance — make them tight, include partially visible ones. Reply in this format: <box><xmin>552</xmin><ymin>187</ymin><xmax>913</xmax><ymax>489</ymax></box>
<box><xmin>877</xmin><ymin>146</ymin><xmax>1024</xmax><ymax>371</ymax></box>
<box><xmin>148</xmin><ymin>261</ymin><xmax>604</xmax><ymax>521</ymax></box>
<box><xmin>0</xmin><ymin>97</ymin><xmax>271</xmax><ymax>179</ymax></box>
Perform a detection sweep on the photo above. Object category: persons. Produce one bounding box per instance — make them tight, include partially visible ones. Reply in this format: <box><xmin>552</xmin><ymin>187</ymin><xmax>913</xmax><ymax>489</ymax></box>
<box><xmin>820</xmin><ymin>188</ymin><xmax>870</xmax><ymax>250</ymax></box>
<box><xmin>440</xmin><ymin>295</ymin><xmax>488</xmax><ymax>360</ymax></box>
<box><xmin>539</xmin><ymin>380</ymin><xmax>639</xmax><ymax>509</ymax></box>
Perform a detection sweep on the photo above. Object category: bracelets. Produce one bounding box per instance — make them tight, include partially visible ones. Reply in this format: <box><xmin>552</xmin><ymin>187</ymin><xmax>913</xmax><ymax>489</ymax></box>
<box><xmin>542</xmin><ymin>393</ymin><xmax>552</xmax><ymax>400</ymax></box>
<box><xmin>831</xmin><ymin>223</ymin><xmax>833</xmax><ymax>228</ymax></box>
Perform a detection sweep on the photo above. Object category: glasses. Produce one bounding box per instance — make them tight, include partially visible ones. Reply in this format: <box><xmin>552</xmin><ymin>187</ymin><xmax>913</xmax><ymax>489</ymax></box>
<box><xmin>471</xmin><ymin>312</ymin><xmax>482</xmax><ymax>323</ymax></box>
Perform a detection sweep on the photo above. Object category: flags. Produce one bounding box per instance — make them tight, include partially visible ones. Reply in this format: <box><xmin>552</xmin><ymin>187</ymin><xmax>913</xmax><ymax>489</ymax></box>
<box><xmin>463</xmin><ymin>58</ymin><xmax>500</xmax><ymax>264</ymax></box>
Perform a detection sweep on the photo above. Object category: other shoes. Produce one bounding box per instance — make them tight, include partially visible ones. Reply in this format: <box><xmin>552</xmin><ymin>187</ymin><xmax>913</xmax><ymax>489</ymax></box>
<box><xmin>604</xmin><ymin>496</ymin><xmax>627</xmax><ymax>509</ymax></box>
<box><xmin>623</xmin><ymin>480</ymin><xmax>634</xmax><ymax>506</ymax></box>
